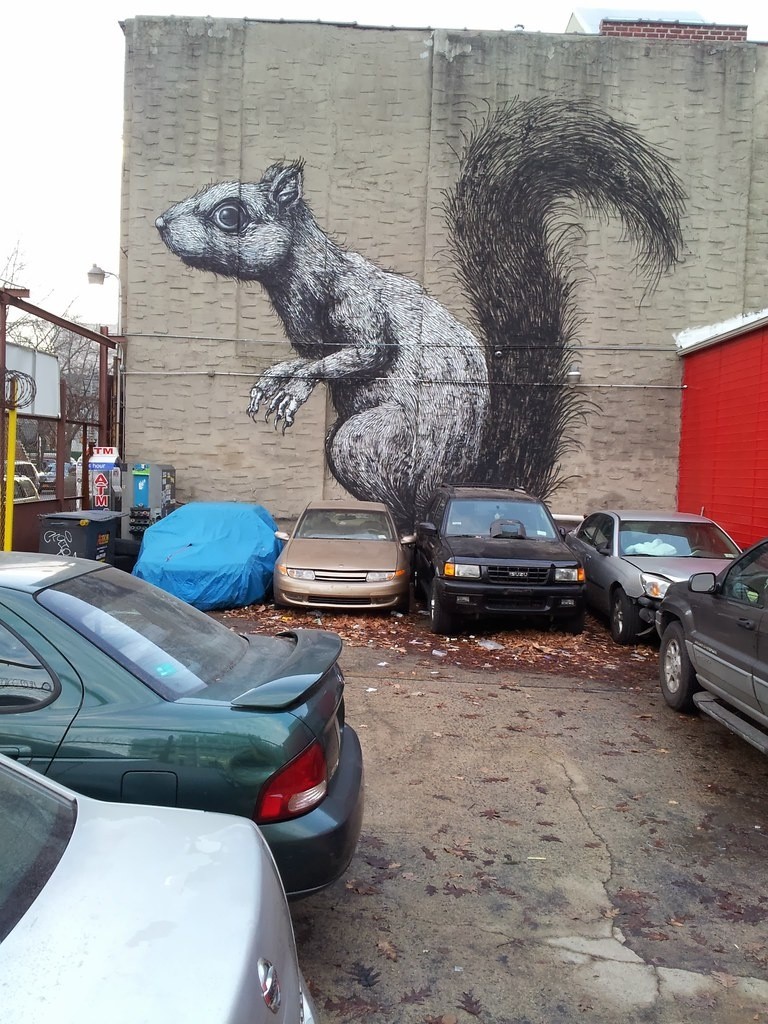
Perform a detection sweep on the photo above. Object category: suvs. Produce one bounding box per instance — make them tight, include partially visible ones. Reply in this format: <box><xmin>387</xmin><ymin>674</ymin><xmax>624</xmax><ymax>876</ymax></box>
<box><xmin>409</xmin><ymin>483</ymin><xmax>589</xmax><ymax>635</ymax></box>
<box><xmin>29</xmin><ymin>458</ymin><xmax>56</xmax><ymax>472</ymax></box>
<box><xmin>656</xmin><ymin>536</ymin><xmax>768</xmax><ymax>757</ymax></box>
<box><xmin>2</xmin><ymin>474</ymin><xmax>40</xmax><ymax>504</ymax></box>
<box><xmin>3</xmin><ymin>459</ymin><xmax>41</xmax><ymax>492</ymax></box>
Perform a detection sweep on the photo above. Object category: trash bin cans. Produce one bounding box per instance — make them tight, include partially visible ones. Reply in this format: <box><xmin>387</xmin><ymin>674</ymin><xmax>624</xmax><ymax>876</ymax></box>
<box><xmin>36</xmin><ymin>510</ymin><xmax>129</xmax><ymax>564</ymax></box>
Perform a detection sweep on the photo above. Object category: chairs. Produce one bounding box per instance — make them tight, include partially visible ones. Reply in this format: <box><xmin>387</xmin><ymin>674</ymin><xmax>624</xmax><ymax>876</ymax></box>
<box><xmin>607</xmin><ymin>526</ymin><xmax>641</xmax><ymax>549</ymax></box>
<box><xmin>360</xmin><ymin>521</ymin><xmax>382</xmax><ymax>534</ymax></box>
<box><xmin>310</xmin><ymin>520</ymin><xmax>337</xmax><ymax>530</ymax></box>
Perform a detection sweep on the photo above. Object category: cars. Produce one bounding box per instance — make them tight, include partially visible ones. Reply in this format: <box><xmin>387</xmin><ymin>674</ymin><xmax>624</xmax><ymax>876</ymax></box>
<box><xmin>563</xmin><ymin>509</ymin><xmax>768</xmax><ymax>645</ymax></box>
<box><xmin>272</xmin><ymin>501</ymin><xmax>416</xmax><ymax>617</ymax></box>
<box><xmin>39</xmin><ymin>462</ymin><xmax>71</xmax><ymax>493</ymax></box>
<box><xmin>0</xmin><ymin>756</ymin><xmax>322</xmax><ymax>1023</ymax></box>
<box><xmin>0</xmin><ymin>547</ymin><xmax>366</xmax><ymax>905</ymax></box>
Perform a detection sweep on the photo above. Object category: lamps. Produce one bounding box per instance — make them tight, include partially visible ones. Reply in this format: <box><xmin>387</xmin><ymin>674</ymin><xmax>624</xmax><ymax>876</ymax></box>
<box><xmin>87</xmin><ymin>264</ymin><xmax>119</xmax><ymax>286</ymax></box>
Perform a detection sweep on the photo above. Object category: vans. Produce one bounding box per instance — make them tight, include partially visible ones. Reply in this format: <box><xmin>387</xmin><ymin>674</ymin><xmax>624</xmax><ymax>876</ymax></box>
<box><xmin>26</xmin><ymin>452</ymin><xmax>76</xmax><ymax>466</ymax></box>
